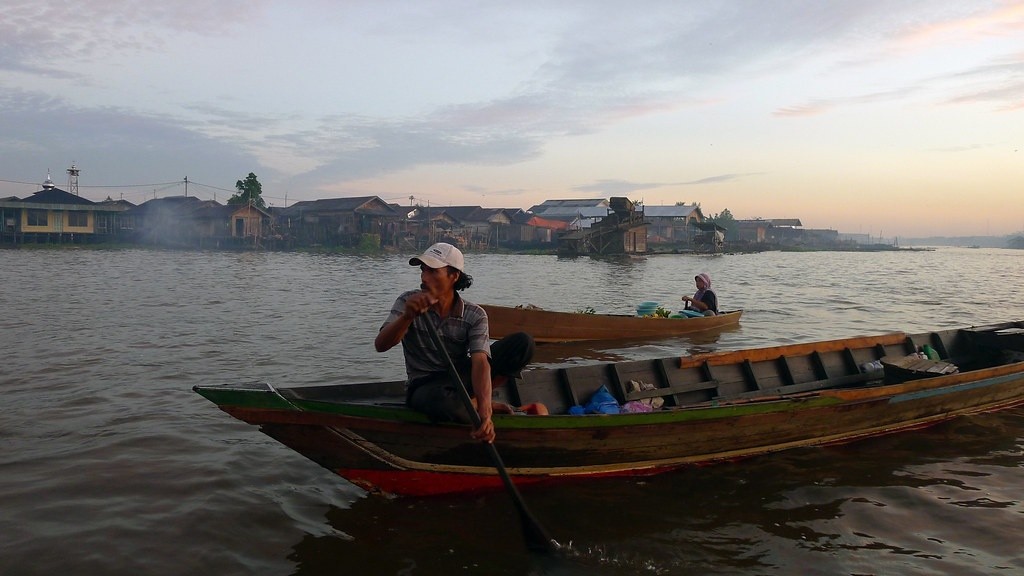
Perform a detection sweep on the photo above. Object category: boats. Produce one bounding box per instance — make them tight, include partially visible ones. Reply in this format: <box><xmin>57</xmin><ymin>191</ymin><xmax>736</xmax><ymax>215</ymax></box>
<box><xmin>190</xmin><ymin>319</ymin><xmax>1024</xmax><ymax>504</ymax></box>
<box><xmin>475</xmin><ymin>304</ymin><xmax>743</xmax><ymax>342</ymax></box>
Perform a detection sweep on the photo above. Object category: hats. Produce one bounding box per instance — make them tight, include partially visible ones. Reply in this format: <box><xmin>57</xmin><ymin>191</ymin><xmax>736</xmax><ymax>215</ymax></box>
<box><xmin>695</xmin><ymin>273</ymin><xmax>711</xmax><ymax>289</ymax></box>
<box><xmin>409</xmin><ymin>242</ymin><xmax>464</xmax><ymax>272</ymax></box>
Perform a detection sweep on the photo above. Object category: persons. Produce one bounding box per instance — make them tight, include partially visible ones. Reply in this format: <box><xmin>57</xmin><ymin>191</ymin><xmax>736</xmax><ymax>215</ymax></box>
<box><xmin>375</xmin><ymin>243</ymin><xmax>536</xmax><ymax>444</ymax></box>
<box><xmin>681</xmin><ymin>274</ymin><xmax>719</xmax><ymax>318</ymax></box>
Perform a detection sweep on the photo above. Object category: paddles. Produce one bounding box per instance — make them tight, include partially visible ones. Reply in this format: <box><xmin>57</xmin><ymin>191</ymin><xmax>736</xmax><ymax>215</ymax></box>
<box><xmin>421</xmin><ymin>310</ymin><xmax>563</xmax><ymax>551</ymax></box>
